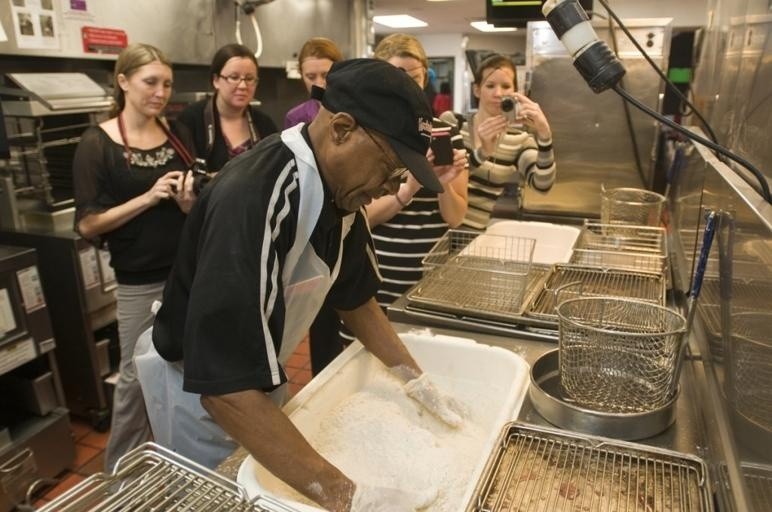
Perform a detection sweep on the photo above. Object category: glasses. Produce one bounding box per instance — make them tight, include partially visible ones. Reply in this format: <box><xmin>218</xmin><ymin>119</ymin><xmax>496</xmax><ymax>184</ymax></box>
<box><xmin>217</xmin><ymin>72</ymin><xmax>262</xmax><ymax>86</ymax></box>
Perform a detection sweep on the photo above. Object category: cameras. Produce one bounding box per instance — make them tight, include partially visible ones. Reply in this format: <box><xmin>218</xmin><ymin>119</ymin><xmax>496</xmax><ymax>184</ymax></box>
<box><xmin>429</xmin><ymin>132</ymin><xmax>454</xmax><ymax>166</ymax></box>
<box><xmin>170</xmin><ymin>162</ymin><xmax>212</xmax><ymax>196</ymax></box>
<box><xmin>501</xmin><ymin>94</ymin><xmax>516</xmax><ymax>124</ymax></box>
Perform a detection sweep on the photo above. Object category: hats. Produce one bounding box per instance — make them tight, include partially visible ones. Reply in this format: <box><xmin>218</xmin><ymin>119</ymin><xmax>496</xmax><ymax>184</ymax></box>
<box><xmin>310</xmin><ymin>58</ymin><xmax>445</xmax><ymax>193</ymax></box>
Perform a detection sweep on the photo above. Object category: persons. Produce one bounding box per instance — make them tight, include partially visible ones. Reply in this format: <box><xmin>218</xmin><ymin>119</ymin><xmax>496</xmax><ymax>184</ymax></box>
<box><xmin>338</xmin><ymin>32</ymin><xmax>470</xmax><ymax>346</ymax></box>
<box><xmin>284</xmin><ymin>36</ymin><xmax>345</xmax><ymax>379</ymax></box>
<box><xmin>70</xmin><ymin>41</ymin><xmax>199</xmax><ymax>473</ymax></box>
<box><xmin>437</xmin><ymin>49</ymin><xmax>557</xmax><ymax>232</ymax></box>
<box><xmin>130</xmin><ymin>53</ymin><xmax>479</xmax><ymax>510</ymax></box>
<box><xmin>432</xmin><ymin>80</ymin><xmax>450</xmax><ymax>118</ymax></box>
<box><xmin>179</xmin><ymin>43</ymin><xmax>275</xmax><ymax>174</ymax></box>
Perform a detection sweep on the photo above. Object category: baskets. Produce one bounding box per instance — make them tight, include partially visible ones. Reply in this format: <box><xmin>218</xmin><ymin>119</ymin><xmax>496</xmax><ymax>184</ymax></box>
<box><xmin>420</xmin><ymin>228</ymin><xmax>537</xmax><ymax>316</ymax></box>
<box><xmin>553</xmin><ymin>296</ymin><xmax>689</xmax><ymax>413</ymax></box>
<box><xmin>572</xmin><ymin>181</ymin><xmax>671</xmax><ymax>275</ymax></box>
